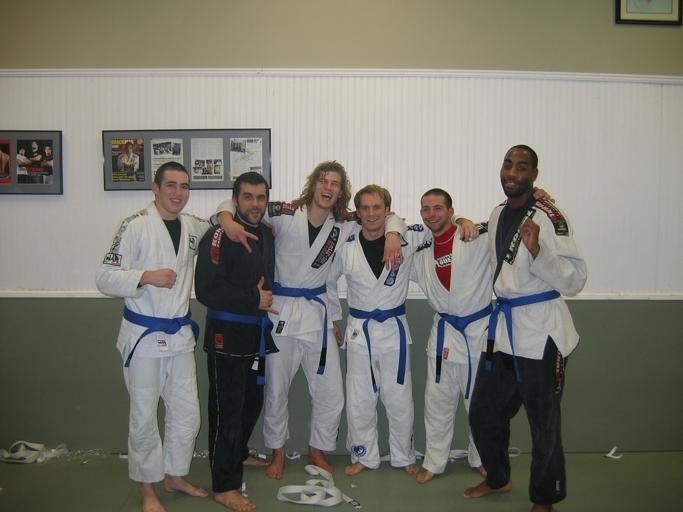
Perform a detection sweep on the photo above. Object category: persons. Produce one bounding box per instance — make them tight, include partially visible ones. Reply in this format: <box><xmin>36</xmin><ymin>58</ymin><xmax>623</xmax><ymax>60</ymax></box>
<box><xmin>0</xmin><ymin>141</ymin><xmax>54</xmax><ymax>184</ymax></box>
<box><xmin>194</xmin><ymin>171</ymin><xmax>278</xmax><ymax>512</ymax></box>
<box><xmin>463</xmin><ymin>145</ymin><xmax>590</xmax><ymax>512</ymax></box>
<box><xmin>117</xmin><ymin>142</ymin><xmax>139</xmax><ymax>172</ymax></box>
<box><xmin>216</xmin><ymin>159</ymin><xmax>406</xmax><ymax>479</ymax></box>
<box><xmin>95</xmin><ymin>161</ymin><xmax>212</xmax><ymax>512</ymax></box>
<box><xmin>410</xmin><ymin>188</ymin><xmax>555</xmax><ymax>482</ymax></box>
<box><xmin>326</xmin><ymin>182</ymin><xmax>480</xmax><ymax>473</ymax></box>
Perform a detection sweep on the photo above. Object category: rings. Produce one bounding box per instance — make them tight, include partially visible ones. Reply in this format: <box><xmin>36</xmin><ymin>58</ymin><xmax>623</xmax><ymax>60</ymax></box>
<box><xmin>396</xmin><ymin>254</ymin><xmax>400</xmax><ymax>258</ymax></box>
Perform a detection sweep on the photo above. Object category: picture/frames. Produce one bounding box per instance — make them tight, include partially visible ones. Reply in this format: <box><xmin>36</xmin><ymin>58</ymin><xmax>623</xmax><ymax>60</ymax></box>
<box><xmin>1</xmin><ymin>130</ymin><xmax>64</xmax><ymax>195</ymax></box>
<box><xmin>103</xmin><ymin>129</ymin><xmax>273</xmax><ymax>190</ymax></box>
<box><xmin>615</xmin><ymin>0</ymin><xmax>683</xmax><ymax>25</ymax></box>
